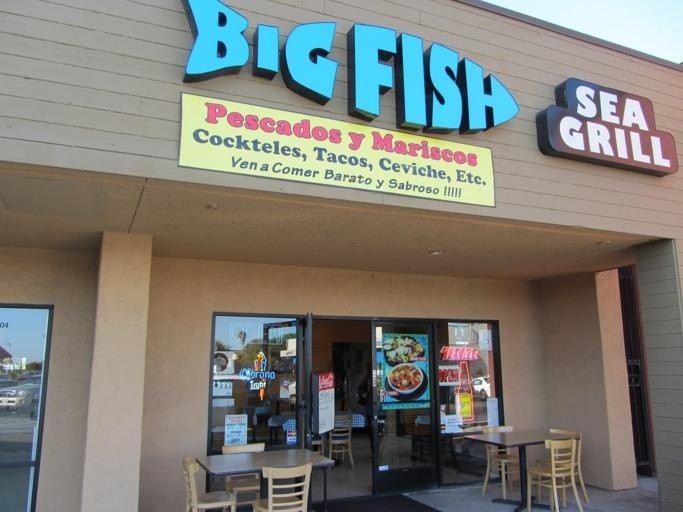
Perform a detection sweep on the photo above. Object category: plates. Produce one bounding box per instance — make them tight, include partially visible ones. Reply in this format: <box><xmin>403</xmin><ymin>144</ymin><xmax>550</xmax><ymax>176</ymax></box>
<box><xmin>381</xmin><ymin>333</ymin><xmax>429</xmax><ymax>401</ymax></box>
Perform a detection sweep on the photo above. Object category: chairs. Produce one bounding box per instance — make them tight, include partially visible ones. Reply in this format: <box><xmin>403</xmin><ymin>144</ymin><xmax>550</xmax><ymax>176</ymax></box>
<box><xmin>220</xmin><ymin>442</ymin><xmax>267</xmax><ymax>512</ymax></box>
<box><xmin>182</xmin><ymin>456</ymin><xmax>237</xmax><ymax>512</ymax></box>
<box><xmin>212</xmin><ymin>392</ymin><xmax>488</xmax><ymax>470</ymax></box>
<box><xmin>251</xmin><ymin>465</ymin><xmax>313</xmax><ymax>511</ymax></box>
<box><xmin>537</xmin><ymin>428</ymin><xmax>589</xmax><ymax>504</ymax></box>
<box><xmin>481</xmin><ymin>425</ymin><xmax>527</xmax><ymax>498</ymax></box>
<box><xmin>528</xmin><ymin>437</ymin><xmax>586</xmax><ymax>512</ymax></box>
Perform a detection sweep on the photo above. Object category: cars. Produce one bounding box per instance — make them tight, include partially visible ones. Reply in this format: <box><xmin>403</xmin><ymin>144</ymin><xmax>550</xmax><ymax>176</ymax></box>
<box><xmin>0</xmin><ymin>371</ymin><xmax>41</xmax><ymax>411</ymax></box>
<box><xmin>471</xmin><ymin>377</ymin><xmax>490</xmax><ymax>401</ymax></box>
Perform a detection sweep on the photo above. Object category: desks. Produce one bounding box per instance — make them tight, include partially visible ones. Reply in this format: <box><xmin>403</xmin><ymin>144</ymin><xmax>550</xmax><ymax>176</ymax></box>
<box><xmin>194</xmin><ymin>449</ymin><xmax>335</xmax><ymax>512</ymax></box>
<box><xmin>464</xmin><ymin>431</ymin><xmax>581</xmax><ymax>512</ymax></box>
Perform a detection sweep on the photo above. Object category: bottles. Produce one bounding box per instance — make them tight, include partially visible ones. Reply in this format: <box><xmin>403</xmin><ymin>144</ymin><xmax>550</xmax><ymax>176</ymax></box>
<box><xmin>455</xmin><ymin>361</ymin><xmax>474</xmax><ymax>424</ymax></box>
